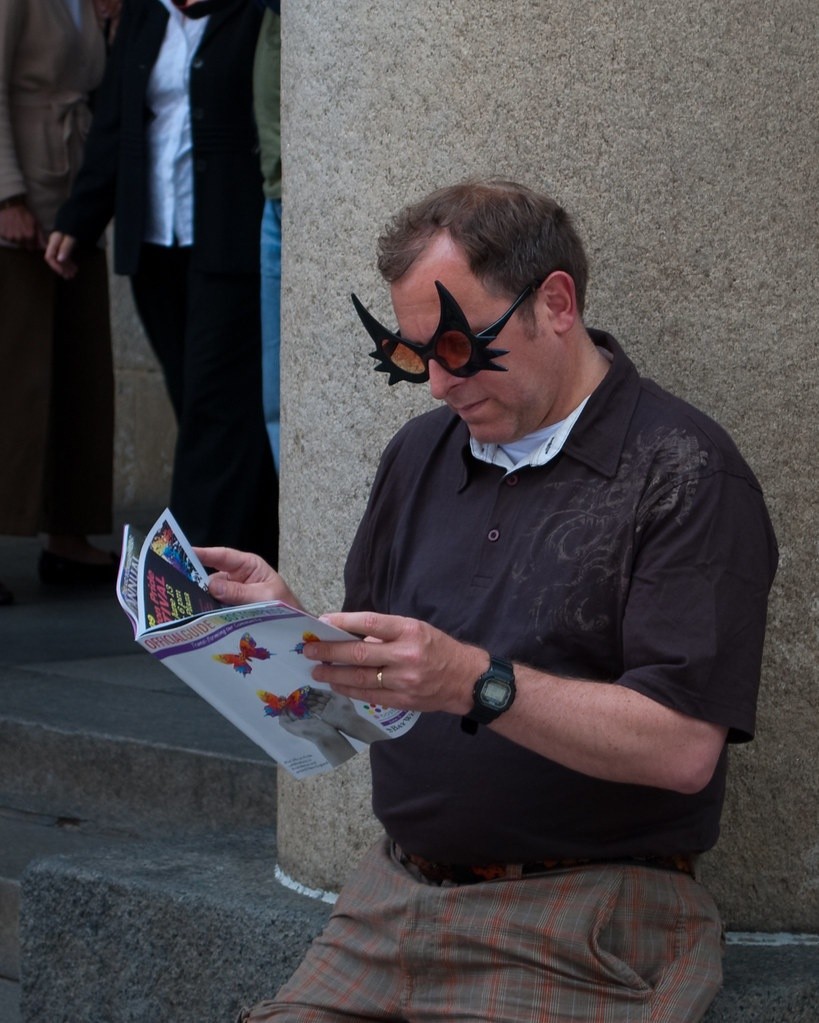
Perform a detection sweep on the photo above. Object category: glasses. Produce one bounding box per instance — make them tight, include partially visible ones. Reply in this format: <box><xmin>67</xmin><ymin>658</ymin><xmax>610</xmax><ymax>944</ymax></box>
<box><xmin>351</xmin><ymin>279</ymin><xmax>544</xmax><ymax>386</ymax></box>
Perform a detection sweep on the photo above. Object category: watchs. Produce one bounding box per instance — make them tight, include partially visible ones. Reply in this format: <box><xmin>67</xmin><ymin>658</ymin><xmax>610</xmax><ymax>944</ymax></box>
<box><xmin>461</xmin><ymin>654</ymin><xmax>517</xmax><ymax>735</ymax></box>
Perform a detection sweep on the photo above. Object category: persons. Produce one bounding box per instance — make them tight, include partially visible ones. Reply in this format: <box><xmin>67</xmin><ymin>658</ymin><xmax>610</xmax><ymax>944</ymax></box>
<box><xmin>237</xmin><ymin>174</ymin><xmax>780</xmax><ymax>1023</ymax></box>
<box><xmin>278</xmin><ymin>685</ymin><xmax>392</xmax><ymax>768</ymax></box>
<box><xmin>0</xmin><ymin>0</ymin><xmax>279</xmax><ymax>603</ymax></box>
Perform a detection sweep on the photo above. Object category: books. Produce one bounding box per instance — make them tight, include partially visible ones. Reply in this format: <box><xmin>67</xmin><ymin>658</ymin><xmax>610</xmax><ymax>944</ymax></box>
<box><xmin>114</xmin><ymin>507</ymin><xmax>424</xmax><ymax>781</ymax></box>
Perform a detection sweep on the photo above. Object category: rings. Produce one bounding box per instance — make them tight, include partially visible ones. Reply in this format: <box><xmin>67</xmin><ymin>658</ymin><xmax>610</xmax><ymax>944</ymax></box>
<box><xmin>376</xmin><ymin>667</ymin><xmax>383</xmax><ymax>690</ymax></box>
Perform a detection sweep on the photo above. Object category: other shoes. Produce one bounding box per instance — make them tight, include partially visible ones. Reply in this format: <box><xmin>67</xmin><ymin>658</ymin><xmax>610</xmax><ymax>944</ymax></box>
<box><xmin>37</xmin><ymin>547</ymin><xmax>121</xmax><ymax>590</ymax></box>
<box><xmin>0</xmin><ymin>584</ymin><xmax>14</xmax><ymax>606</ymax></box>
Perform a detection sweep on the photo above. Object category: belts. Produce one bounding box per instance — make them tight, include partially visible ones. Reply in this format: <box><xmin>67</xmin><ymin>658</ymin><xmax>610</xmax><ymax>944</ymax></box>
<box><xmin>384</xmin><ymin>833</ymin><xmax>696</xmax><ymax>888</ymax></box>
